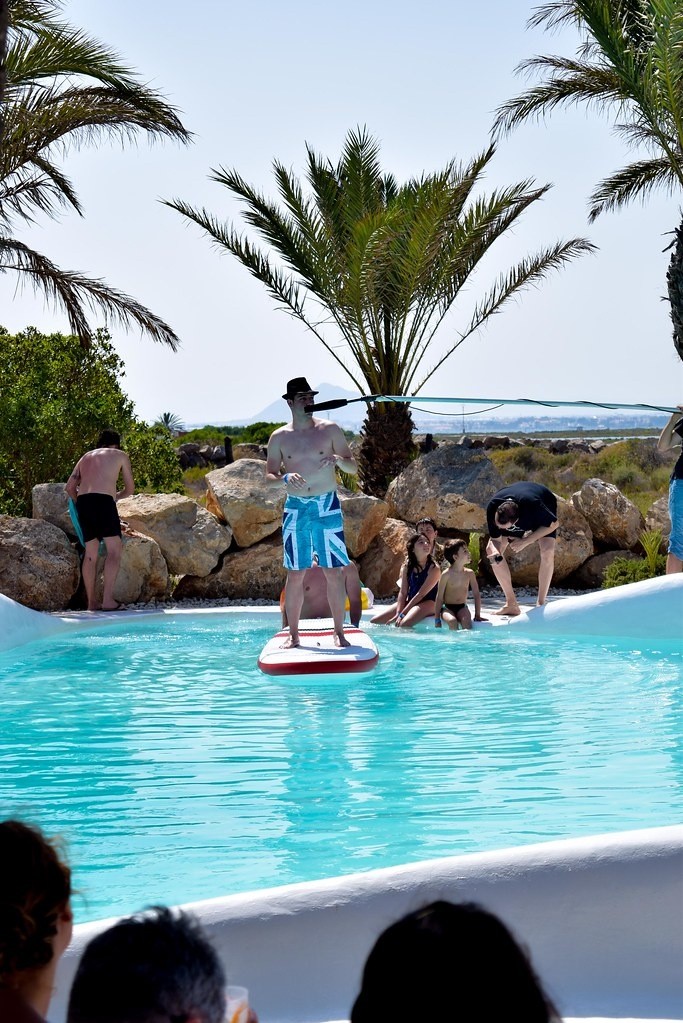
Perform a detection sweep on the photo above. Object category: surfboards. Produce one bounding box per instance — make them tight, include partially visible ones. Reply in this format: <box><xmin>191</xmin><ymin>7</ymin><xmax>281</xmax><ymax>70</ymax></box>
<box><xmin>256</xmin><ymin>616</ymin><xmax>381</xmax><ymax>677</ymax></box>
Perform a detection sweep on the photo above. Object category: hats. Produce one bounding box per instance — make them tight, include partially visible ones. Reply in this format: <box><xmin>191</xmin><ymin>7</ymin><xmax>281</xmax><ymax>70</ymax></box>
<box><xmin>282</xmin><ymin>377</ymin><xmax>319</xmax><ymax>400</ymax></box>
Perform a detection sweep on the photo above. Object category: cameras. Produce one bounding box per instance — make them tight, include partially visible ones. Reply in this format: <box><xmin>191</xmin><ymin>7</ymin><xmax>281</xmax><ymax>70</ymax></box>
<box><xmin>494</xmin><ymin>555</ymin><xmax>503</xmax><ymax>564</ymax></box>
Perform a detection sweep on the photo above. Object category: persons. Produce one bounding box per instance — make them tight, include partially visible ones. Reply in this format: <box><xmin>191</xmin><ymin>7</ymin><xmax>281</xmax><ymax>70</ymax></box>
<box><xmin>370</xmin><ymin>517</ymin><xmax>451</xmax><ymax>628</ymax></box>
<box><xmin>282</xmin><ymin>554</ymin><xmax>362</xmax><ymax>628</ymax></box>
<box><xmin>435</xmin><ymin>539</ymin><xmax>489</xmax><ymax>631</ymax></box>
<box><xmin>0</xmin><ymin>818</ymin><xmax>562</xmax><ymax>1023</ymax></box>
<box><xmin>657</xmin><ymin>405</ymin><xmax>683</xmax><ymax>575</ymax></box>
<box><xmin>264</xmin><ymin>377</ymin><xmax>359</xmax><ymax>649</ymax></box>
<box><xmin>65</xmin><ymin>431</ymin><xmax>134</xmax><ymax>611</ymax></box>
<box><xmin>485</xmin><ymin>481</ymin><xmax>560</xmax><ymax>615</ymax></box>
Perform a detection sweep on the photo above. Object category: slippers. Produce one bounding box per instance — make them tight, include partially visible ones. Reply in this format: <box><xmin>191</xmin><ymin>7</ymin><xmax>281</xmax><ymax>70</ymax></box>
<box><xmin>101</xmin><ymin>599</ymin><xmax>129</xmax><ymax>610</ymax></box>
<box><xmin>90</xmin><ymin>608</ymin><xmax>103</xmax><ymax>611</ymax></box>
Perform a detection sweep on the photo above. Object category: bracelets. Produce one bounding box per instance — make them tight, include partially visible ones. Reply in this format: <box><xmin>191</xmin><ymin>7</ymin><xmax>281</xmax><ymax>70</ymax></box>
<box><xmin>283</xmin><ymin>472</ymin><xmax>289</xmax><ymax>485</ymax></box>
<box><xmin>352</xmin><ymin>622</ymin><xmax>359</xmax><ymax>628</ymax></box>
<box><xmin>399</xmin><ymin>609</ymin><xmax>407</xmax><ymax>618</ymax></box>
<box><xmin>435</xmin><ymin>619</ymin><xmax>441</xmax><ymax>624</ymax></box>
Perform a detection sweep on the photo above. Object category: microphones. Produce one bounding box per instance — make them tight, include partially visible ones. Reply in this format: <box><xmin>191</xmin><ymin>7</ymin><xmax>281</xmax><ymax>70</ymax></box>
<box><xmin>304</xmin><ymin>395</ymin><xmax>377</xmax><ymax>413</ymax></box>
